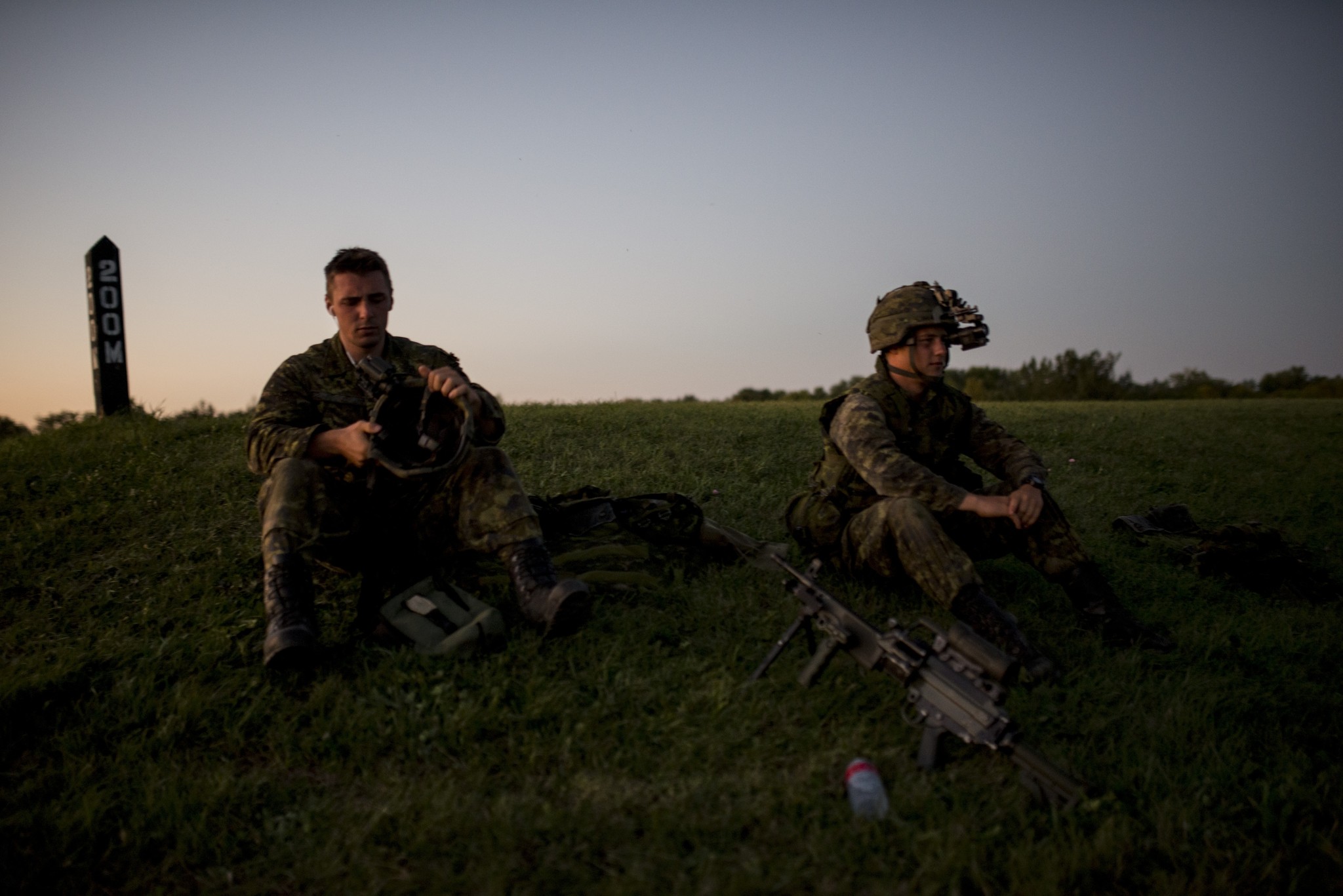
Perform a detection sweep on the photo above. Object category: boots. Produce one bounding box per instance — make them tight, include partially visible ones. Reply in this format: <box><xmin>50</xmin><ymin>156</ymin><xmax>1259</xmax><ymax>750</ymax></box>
<box><xmin>503</xmin><ymin>543</ymin><xmax>591</xmax><ymax>641</ymax></box>
<box><xmin>258</xmin><ymin>554</ymin><xmax>326</xmax><ymax>671</ymax></box>
<box><xmin>1051</xmin><ymin>556</ymin><xmax>1178</xmax><ymax>654</ymax></box>
<box><xmin>944</xmin><ymin>582</ymin><xmax>1063</xmax><ymax>696</ymax></box>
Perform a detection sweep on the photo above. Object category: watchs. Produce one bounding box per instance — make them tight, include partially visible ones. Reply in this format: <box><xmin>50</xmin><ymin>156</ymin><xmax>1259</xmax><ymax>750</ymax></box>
<box><xmin>1023</xmin><ymin>476</ymin><xmax>1045</xmax><ymax>491</ymax></box>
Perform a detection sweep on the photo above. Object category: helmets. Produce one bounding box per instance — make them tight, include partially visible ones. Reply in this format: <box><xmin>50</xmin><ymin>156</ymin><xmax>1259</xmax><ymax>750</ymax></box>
<box><xmin>368</xmin><ymin>376</ymin><xmax>477</xmax><ymax>480</ymax></box>
<box><xmin>867</xmin><ymin>281</ymin><xmax>955</xmax><ymax>354</ymax></box>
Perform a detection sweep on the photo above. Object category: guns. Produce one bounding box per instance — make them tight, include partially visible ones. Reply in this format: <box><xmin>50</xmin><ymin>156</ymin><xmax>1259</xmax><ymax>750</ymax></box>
<box><xmin>748</xmin><ymin>549</ymin><xmax>1087</xmax><ymax>810</ymax></box>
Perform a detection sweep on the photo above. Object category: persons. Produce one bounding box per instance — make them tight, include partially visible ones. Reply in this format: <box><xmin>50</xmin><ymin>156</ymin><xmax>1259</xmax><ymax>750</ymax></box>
<box><xmin>245</xmin><ymin>245</ymin><xmax>593</xmax><ymax>666</ymax></box>
<box><xmin>783</xmin><ymin>282</ymin><xmax>1171</xmax><ymax>680</ymax></box>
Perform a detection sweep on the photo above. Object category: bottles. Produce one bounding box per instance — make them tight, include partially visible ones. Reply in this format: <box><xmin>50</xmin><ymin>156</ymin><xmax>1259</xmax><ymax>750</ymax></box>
<box><xmin>846</xmin><ymin>755</ymin><xmax>888</xmax><ymax>821</ymax></box>
